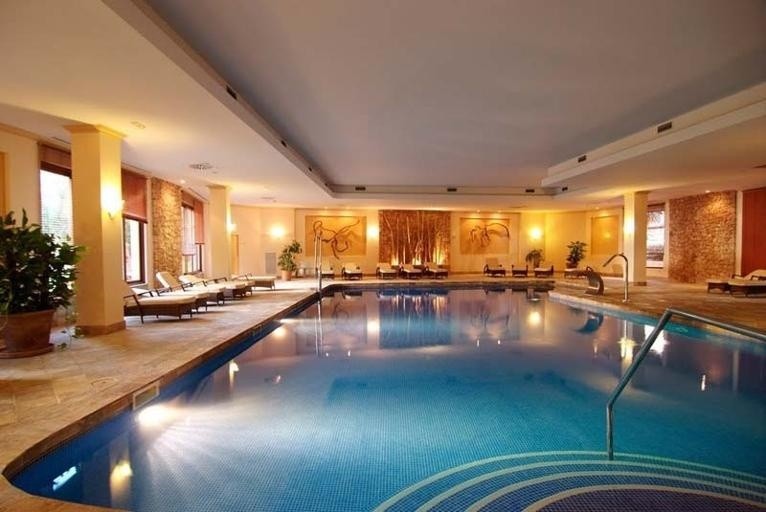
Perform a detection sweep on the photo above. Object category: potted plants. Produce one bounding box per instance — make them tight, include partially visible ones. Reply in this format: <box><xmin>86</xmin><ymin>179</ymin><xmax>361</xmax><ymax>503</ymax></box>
<box><xmin>563</xmin><ymin>240</ymin><xmax>587</xmax><ymax>267</ymax></box>
<box><xmin>278</xmin><ymin>239</ymin><xmax>302</xmax><ymax>280</ymax></box>
<box><xmin>0</xmin><ymin>207</ymin><xmax>90</xmax><ymax>359</ymax></box>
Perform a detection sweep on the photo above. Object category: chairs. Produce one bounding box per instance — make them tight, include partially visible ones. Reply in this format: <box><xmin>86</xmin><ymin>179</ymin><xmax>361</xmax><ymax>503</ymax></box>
<box><xmin>118</xmin><ymin>270</ymin><xmax>277</xmax><ymax>323</ymax></box>
<box><xmin>317</xmin><ymin>261</ymin><xmax>449</xmax><ymax>280</ymax></box>
<box><xmin>704</xmin><ymin>269</ymin><xmax>766</xmax><ymax>297</ymax></box>
<box><xmin>482</xmin><ymin>257</ymin><xmax>588</xmax><ymax>277</ymax></box>
